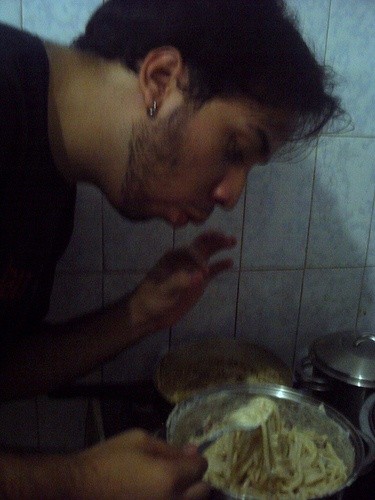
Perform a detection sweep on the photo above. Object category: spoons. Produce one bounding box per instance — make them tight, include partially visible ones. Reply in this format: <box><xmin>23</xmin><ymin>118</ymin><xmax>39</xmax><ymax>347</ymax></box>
<box><xmin>194</xmin><ymin>397</ymin><xmax>274</xmax><ymax>454</ymax></box>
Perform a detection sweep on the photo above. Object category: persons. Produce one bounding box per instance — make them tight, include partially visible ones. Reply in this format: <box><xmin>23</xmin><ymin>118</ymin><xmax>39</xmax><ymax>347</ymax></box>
<box><xmin>0</xmin><ymin>0</ymin><xmax>356</xmax><ymax>500</ymax></box>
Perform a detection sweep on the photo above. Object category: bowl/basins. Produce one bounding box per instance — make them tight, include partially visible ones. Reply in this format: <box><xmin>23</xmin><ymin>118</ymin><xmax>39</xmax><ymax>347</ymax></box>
<box><xmin>359</xmin><ymin>392</ymin><xmax>375</xmax><ymax>445</ymax></box>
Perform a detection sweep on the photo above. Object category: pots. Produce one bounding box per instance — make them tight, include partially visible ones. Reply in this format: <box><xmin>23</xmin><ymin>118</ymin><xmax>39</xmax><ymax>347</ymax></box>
<box><xmin>47</xmin><ymin>337</ymin><xmax>297</xmax><ymax>414</ymax></box>
<box><xmin>164</xmin><ymin>382</ymin><xmax>375</xmax><ymax>500</ymax></box>
<box><xmin>293</xmin><ymin>329</ymin><xmax>375</xmax><ymax>430</ymax></box>
